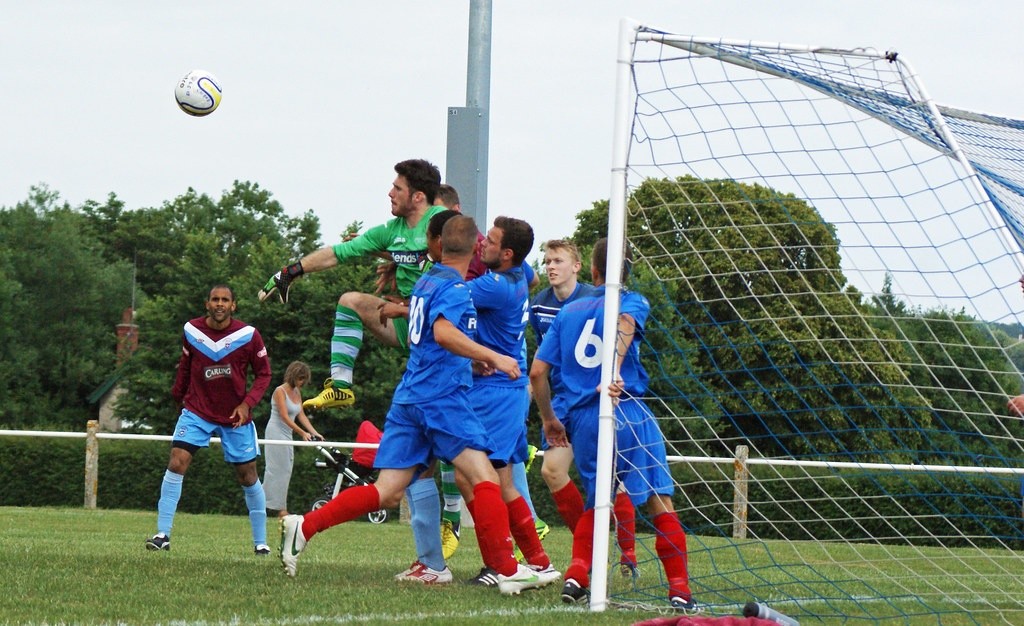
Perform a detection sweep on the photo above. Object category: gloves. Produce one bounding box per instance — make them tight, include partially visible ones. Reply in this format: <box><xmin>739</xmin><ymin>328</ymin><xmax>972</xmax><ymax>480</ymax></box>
<box><xmin>258</xmin><ymin>262</ymin><xmax>304</xmax><ymax>304</ymax></box>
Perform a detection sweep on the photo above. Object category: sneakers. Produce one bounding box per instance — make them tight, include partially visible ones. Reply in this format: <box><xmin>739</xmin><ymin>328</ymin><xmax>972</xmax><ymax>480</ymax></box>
<box><xmin>620</xmin><ymin>564</ymin><xmax>648</xmax><ymax>586</ymax></box>
<box><xmin>277</xmin><ymin>515</ymin><xmax>307</xmax><ymax>578</ymax></box>
<box><xmin>497</xmin><ymin>564</ymin><xmax>552</xmax><ymax>597</ymax></box>
<box><xmin>524</xmin><ymin>445</ymin><xmax>538</xmax><ymax>474</ymax></box>
<box><xmin>467</xmin><ymin>565</ymin><xmax>498</xmax><ymax>588</ymax></box>
<box><xmin>441</xmin><ymin>517</ymin><xmax>460</xmax><ymax>559</ymax></box>
<box><xmin>561</xmin><ymin>579</ymin><xmax>590</xmax><ymax>611</ymax></box>
<box><xmin>146</xmin><ymin>532</ymin><xmax>169</xmax><ymax>553</ymax></box>
<box><xmin>514</xmin><ymin>520</ymin><xmax>549</xmax><ymax>563</ymax></box>
<box><xmin>254</xmin><ymin>543</ymin><xmax>270</xmax><ymax>557</ymax></box>
<box><xmin>395</xmin><ymin>560</ymin><xmax>452</xmax><ymax>584</ymax></box>
<box><xmin>302</xmin><ymin>378</ymin><xmax>355</xmax><ymax>411</ymax></box>
<box><xmin>671</xmin><ymin>596</ymin><xmax>704</xmax><ymax>615</ymax></box>
<box><xmin>528</xmin><ymin>564</ymin><xmax>562</xmax><ymax>585</ymax></box>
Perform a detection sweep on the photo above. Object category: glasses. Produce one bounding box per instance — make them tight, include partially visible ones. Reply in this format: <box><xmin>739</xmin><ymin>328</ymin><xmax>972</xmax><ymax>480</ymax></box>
<box><xmin>296</xmin><ymin>375</ymin><xmax>305</xmax><ymax>380</ymax></box>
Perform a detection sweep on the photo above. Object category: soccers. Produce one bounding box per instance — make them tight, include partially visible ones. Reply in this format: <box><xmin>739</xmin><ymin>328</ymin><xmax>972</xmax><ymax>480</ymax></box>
<box><xmin>174</xmin><ymin>68</ymin><xmax>223</xmax><ymax>117</ymax></box>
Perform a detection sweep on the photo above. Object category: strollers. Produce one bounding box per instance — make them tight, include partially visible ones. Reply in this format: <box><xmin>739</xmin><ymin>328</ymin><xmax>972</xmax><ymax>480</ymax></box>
<box><xmin>306</xmin><ymin>419</ymin><xmax>395</xmax><ymax>524</ymax></box>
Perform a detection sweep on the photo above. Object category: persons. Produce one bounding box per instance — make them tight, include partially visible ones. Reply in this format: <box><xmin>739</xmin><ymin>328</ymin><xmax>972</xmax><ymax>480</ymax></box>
<box><xmin>258</xmin><ymin>159</ymin><xmax>550</xmax><ymax>587</ymax></box>
<box><xmin>260</xmin><ymin>361</ymin><xmax>323</xmax><ymax>517</ymax></box>
<box><xmin>529</xmin><ymin>237</ymin><xmax>700</xmax><ymax>612</ymax></box>
<box><xmin>376</xmin><ymin>216</ymin><xmax>562</xmax><ymax>583</ymax></box>
<box><xmin>145</xmin><ymin>284</ymin><xmax>271</xmax><ymax>554</ymax></box>
<box><xmin>528</xmin><ymin>239</ymin><xmax>640</xmax><ymax>578</ymax></box>
<box><xmin>277</xmin><ymin>215</ymin><xmax>552</xmax><ymax>596</ymax></box>
